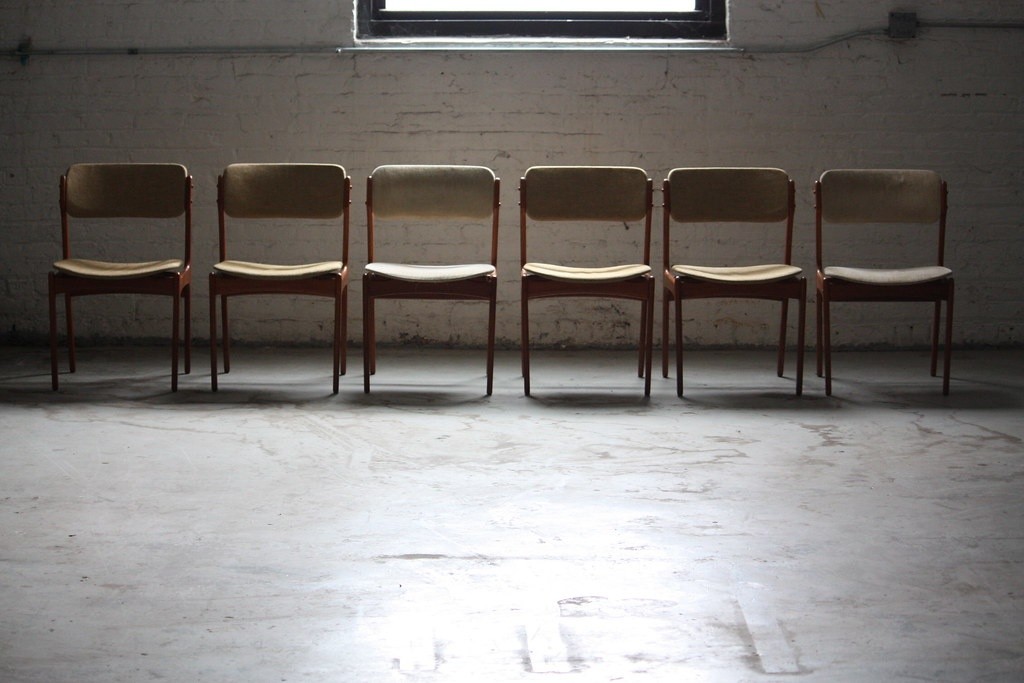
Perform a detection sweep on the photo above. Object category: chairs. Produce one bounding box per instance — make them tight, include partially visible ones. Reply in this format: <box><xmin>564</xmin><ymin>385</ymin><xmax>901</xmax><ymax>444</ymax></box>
<box><xmin>208</xmin><ymin>162</ymin><xmax>352</xmax><ymax>394</ymax></box>
<box><xmin>46</xmin><ymin>161</ymin><xmax>195</xmax><ymax>392</ymax></box>
<box><xmin>516</xmin><ymin>165</ymin><xmax>655</xmax><ymax>397</ymax></box>
<box><xmin>361</xmin><ymin>164</ymin><xmax>502</xmax><ymax>395</ymax></box>
<box><xmin>812</xmin><ymin>167</ymin><xmax>956</xmax><ymax>397</ymax></box>
<box><xmin>660</xmin><ymin>166</ymin><xmax>808</xmax><ymax>396</ymax></box>
